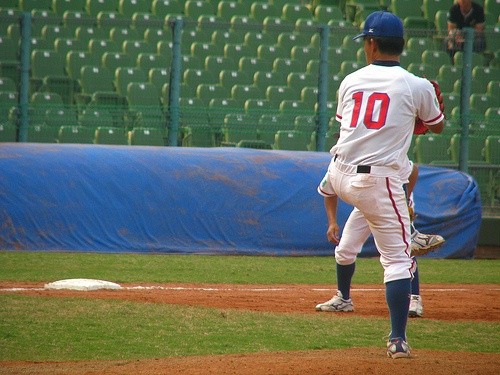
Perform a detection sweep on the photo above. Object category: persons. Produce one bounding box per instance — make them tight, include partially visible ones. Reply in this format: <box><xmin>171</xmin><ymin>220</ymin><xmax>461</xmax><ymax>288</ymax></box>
<box><xmin>316</xmin><ymin>161</ymin><xmax>424</xmax><ymax>317</ymax></box>
<box><xmin>446</xmin><ymin>0</ymin><xmax>486</xmax><ymax>64</ymax></box>
<box><xmin>328</xmin><ymin>10</ymin><xmax>444</xmax><ymax>359</ymax></box>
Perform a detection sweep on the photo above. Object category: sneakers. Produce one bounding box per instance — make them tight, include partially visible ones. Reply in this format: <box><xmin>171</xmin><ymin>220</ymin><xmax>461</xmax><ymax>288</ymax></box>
<box><xmin>410</xmin><ymin>230</ymin><xmax>446</xmax><ymax>257</ymax></box>
<box><xmin>315</xmin><ymin>290</ymin><xmax>355</xmax><ymax>311</ymax></box>
<box><xmin>382</xmin><ymin>331</ymin><xmax>410</xmax><ymax>358</ymax></box>
<box><xmin>408</xmin><ymin>295</ymin><xmax>423</xmax><ymax>317</ymax></box>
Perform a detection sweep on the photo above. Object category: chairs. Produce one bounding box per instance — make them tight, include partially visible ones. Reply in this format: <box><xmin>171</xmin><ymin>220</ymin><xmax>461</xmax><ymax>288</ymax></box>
<box><xmin>0</xmin><ymin>0</ymin><xmax>500</xmax><ymax>206</ymax></box>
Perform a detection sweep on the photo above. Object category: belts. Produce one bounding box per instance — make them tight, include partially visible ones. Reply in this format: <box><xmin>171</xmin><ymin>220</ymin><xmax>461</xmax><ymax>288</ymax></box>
<box><xmin>334</xmin><ymin>154</ymin><xmax>370</xmax><ymax>173</ymax></box>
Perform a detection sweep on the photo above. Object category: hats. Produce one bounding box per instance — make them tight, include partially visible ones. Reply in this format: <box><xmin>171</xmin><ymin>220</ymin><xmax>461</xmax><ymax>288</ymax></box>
<box><xmin>353</xmin><ymin>10</ymin><xmax>403</xmax><ymax>40</ymax></box>
